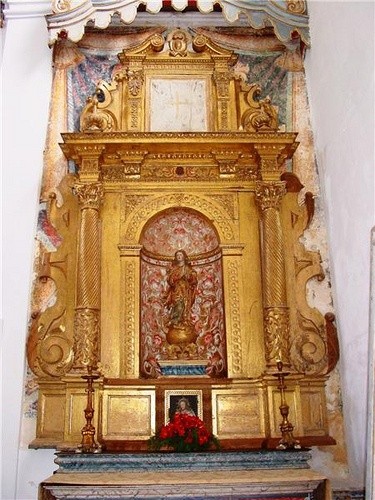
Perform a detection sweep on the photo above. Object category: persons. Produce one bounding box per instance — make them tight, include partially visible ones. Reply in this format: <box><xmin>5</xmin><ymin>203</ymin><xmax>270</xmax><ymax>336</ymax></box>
<box><xmin>164</xmin><ymin>250</ymin><xmax>199</xmax><ymax>327</ymax></box>
<box><xmin>174</xmin><ymin>398</ymin><xmax>195</xmax><ymax>417</ymax></box>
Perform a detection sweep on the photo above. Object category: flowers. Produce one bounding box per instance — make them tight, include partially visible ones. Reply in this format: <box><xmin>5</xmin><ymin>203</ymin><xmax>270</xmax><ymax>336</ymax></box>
<box><xmin>146</xmin><ymin>411</ymin><xmax>224</xmax><ymax>454</ymax></box>
